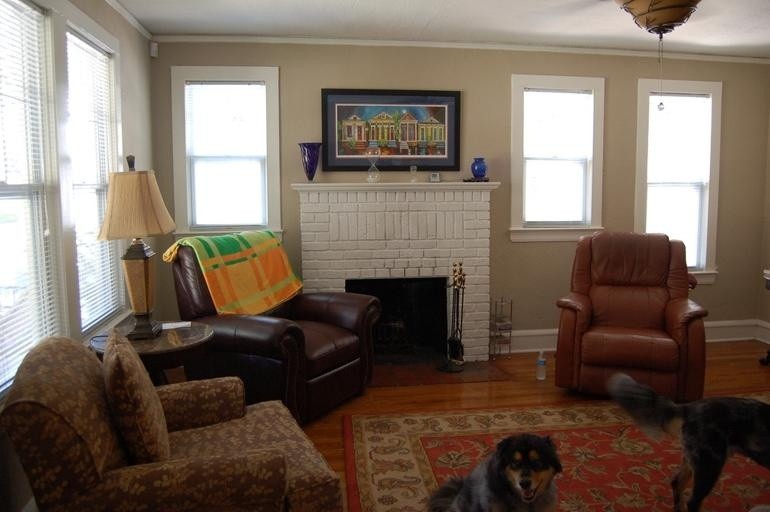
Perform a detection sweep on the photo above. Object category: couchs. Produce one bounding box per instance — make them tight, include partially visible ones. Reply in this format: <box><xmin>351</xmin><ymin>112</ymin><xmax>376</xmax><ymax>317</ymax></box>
<box><xmin>552</xmin><ymin>230</ymin><xmax>708</xmax><ymax>402</ymax></box>
<box><xmin>163</xmin><ymin>227</ymin><xmax>383</xmax><ymax>428</ymax></box>
<box><xmin>1</xmin><ymin>336</ymin><xmax>342</xmax><ymax>510</ymax></box>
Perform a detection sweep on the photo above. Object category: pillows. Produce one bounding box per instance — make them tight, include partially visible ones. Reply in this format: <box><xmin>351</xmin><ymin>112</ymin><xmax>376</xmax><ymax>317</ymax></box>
<box><xmin>100</xmin><ymin>331</ymin><xmax>172</xmax><ymax>462</ymax></box>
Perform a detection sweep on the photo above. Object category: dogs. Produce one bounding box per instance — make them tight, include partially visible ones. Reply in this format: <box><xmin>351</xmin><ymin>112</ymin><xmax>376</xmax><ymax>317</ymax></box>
<box><xmin>606</xmin><ymin>371</ymin><xmax>770</xmax><ymax>512</ymax></box>
<box><xmin>426</xmin><ymin>433</ymin><xmax>562</xmax><ymax>512</ymax></box>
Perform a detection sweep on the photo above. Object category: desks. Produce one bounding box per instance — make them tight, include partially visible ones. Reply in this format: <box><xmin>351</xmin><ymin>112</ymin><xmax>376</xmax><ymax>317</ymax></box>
<box><xmin>88</xmin><ymin>319</ymin><xmax>215</xmax><ymax>383</ymax></box>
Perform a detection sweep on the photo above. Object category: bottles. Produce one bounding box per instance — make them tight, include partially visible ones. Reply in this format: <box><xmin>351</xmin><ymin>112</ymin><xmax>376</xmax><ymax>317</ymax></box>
<box><xmin>410</xmin><ymin>165</ymin><xmax>417</xmax><ymax>183</ymax></box>
<box><xmin>536</xmin><ymin>350</ymin><xmax>546</xmax><ymax>380</ymax></box>
<box><xmin>471</xmin><ymin>157</ymin><xmax>487</xmax><ymax>178</ymax></box>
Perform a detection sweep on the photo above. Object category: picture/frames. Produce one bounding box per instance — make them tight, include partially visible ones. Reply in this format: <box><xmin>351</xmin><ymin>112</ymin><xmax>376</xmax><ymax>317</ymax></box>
<box><xmin>319</xmin><ymin>86</ymin><xmax>462</xmax><ymax>173</ymax></box>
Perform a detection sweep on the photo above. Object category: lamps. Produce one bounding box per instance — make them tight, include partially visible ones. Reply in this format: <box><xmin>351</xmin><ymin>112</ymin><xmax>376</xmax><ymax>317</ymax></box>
<box><xmin>95</xmin><ymin>154</ymin><xmax>178</xmax><ymax>341</ymax></box>
<box><xmin>614</xmin><ymin>1</ymin><xmax>704</xmax><ymax>113</ymax></box>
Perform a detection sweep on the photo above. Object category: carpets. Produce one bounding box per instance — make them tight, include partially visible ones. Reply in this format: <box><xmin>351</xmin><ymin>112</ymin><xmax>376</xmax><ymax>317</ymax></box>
<box><xmin>338</xmin><ymin>395</ymin><xmax>769</xmax><ymax>510</ymax></box>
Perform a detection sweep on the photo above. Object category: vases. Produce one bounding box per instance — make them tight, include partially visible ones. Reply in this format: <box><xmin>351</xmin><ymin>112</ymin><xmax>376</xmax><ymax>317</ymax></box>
<box><xmin>463</xmin><ymin>155</ymin><xmax>490</xmax><ymax>182</ymax></box>
